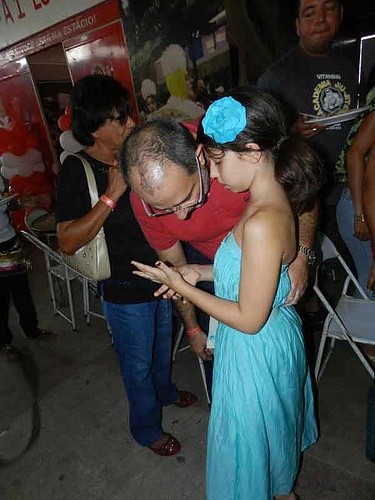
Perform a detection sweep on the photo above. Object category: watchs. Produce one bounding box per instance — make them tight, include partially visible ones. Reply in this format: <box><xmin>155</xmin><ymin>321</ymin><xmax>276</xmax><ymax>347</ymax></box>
<box><xmin>295</xmin><ymin>245</ymin><xmax>315</xmax><ymax>265</ymax></box>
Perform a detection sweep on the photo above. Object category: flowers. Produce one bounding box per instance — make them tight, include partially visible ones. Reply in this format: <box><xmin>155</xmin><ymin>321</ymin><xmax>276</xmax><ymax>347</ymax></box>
<box><xmin>201</xmin><ymin>96</ymin><xmax>247</xmax><ymax>144</ymax></box>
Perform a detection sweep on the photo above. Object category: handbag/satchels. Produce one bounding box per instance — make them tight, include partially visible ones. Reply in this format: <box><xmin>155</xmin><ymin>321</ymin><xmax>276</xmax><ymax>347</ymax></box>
<box><xmin>60</xmin><ymin>153</ymin><xmax>111</xmax><ymax>282</ymax></box>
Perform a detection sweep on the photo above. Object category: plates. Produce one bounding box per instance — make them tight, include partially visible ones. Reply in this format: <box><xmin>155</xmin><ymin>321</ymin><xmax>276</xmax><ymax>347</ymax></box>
<box><xmin>0</xmin><ymin>193</ymin><xmax>18</xmax><ymax>205</ymax></box>
<box><xmin>304</xmin><ymin>105</ymin><xmax>371</xmax><ymax>127</ymax></box>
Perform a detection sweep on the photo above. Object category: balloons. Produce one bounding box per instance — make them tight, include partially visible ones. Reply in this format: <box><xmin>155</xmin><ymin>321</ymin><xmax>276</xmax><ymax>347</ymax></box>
<box><xmin>60</xmin><ymin>151</ymin><xmax>70</xmax><ymax>164</ymax></box>
<box><xmin>60</xmin><ymin>131</ymin><xmax>83</xmax><ymax>153</ymax></box>
<box><xmin>58</xmin><ymin>115</ymin><xmax>71</xmax><ymax>131</ymax></box>
<box><xmin>64</xmin><ymin>106</ymin><xmax>70</xmax><ymax>114</ymax></box>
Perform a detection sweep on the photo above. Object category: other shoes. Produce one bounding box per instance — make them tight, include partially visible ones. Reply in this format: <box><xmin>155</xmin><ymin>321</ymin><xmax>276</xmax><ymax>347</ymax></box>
<box><xmin>149</xmin><ymin>433</ymin><xmax>181</xmax><ymax>456</ymax></box>
<box><xmin>172</xmin><ymin>391</ymin><xmax>196</xmax><ymax>407</ymax></box>
<box><xmin>34</xmin><ymin>330</ymin><xmax>54</xmax><ymax>339</ymax></box>
<box><xmin>1</xmin><ymin>345</ymin><xmax>16</xmax><ymax>361</ymax></box>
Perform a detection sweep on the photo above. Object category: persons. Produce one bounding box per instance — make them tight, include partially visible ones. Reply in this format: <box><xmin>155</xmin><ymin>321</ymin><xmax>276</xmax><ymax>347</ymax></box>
<box><xmin>54</xmin><ymin>0</ymin><xmax>375</xmax><ymax>499</ymax></box>
<box><xmin>0</xmin><ymin>175</ymin><xmax>49</xmax><ymax>354</ymax></box>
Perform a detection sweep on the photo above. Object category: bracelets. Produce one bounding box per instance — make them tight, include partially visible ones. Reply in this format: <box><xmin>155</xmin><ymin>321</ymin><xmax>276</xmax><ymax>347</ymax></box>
<box><xmin>185</xmin><ymin>325</ymin><xmax>201</xmax><ymax>337</ymax></box>
<box><xmin>99</xmin><ymin>194</ymin><xmax>114</xmax><ymax>209</ymax></box>
<box><xmin>352</xmin><ymin>214</ymin><xmax>365</xmax><ymax>222</ymax></box>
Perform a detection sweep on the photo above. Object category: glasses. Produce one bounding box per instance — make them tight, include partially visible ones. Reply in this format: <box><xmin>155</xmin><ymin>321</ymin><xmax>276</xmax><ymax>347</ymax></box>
<box><xmin>104</xmin><ymin>108</ymin><xmax>134</xmax><ymax>125</ymax></box>
<box><xmin>140</xmin><ymin>155</ymin><xmax>203</xmax><ymax>217</ymax></box>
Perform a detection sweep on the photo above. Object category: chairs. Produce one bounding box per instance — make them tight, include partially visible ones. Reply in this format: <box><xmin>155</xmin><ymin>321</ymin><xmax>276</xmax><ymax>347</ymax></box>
<box><xmin>313</xmin><ymin>234</ymin><xmax>374</xmax><ymax>383</ymax></box>
<box><xmin>20</xmin><ymin>206</ymin><xmax>212</xmax><ymax>406</ymax></box>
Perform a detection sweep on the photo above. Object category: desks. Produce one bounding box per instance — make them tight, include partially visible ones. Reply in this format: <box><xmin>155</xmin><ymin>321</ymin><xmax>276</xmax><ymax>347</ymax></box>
<box><xmin>30</xmin><ymin>209</ymin><xmax>57</xmax><ymax>233</ymax></box>
